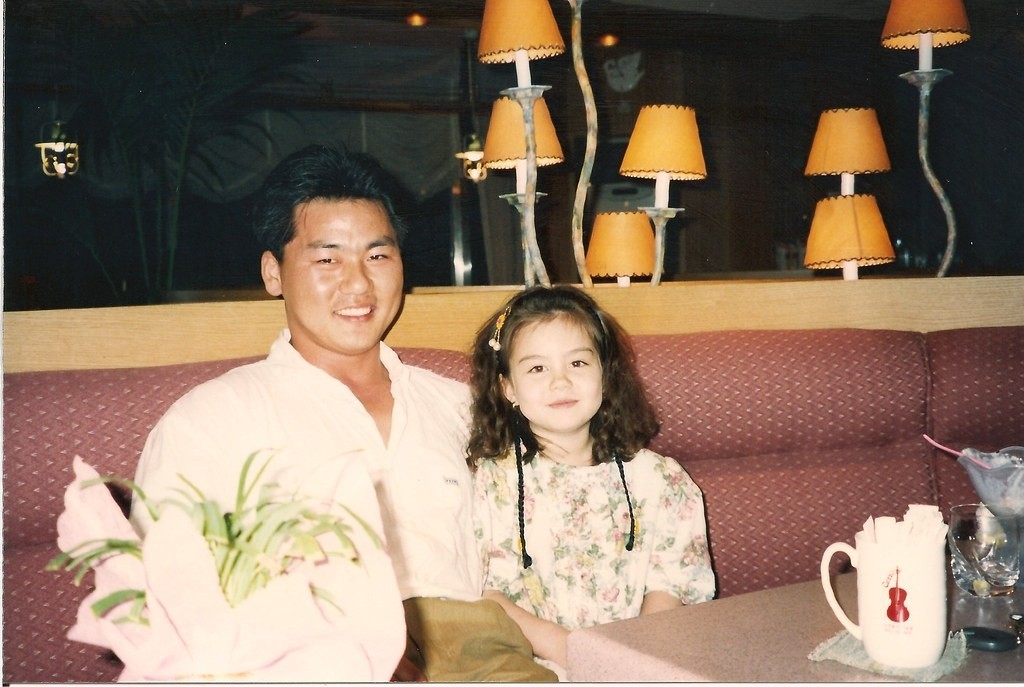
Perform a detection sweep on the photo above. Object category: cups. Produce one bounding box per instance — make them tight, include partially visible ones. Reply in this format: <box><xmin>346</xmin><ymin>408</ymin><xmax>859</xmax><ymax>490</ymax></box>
<box><xmin>819</xmin><ymin>521</ymin><xmax>950</xmax><ymax>669</ymax></box>
<box><xmin>948</xmin><ymin>503</ymin><xmax>1020</xmax><ymax>596</ymax></box>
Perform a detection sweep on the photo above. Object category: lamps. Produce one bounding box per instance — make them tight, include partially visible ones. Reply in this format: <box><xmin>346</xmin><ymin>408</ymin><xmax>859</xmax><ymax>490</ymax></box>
<box><xmin>474</xmin><ymin>0</ymin><xmax>974</xmax><ymax>286</ymax></box>
<box><xmin>34</xmin><ymin>120</ymin><xmax>80</xmax><ymax>178</ymax></box>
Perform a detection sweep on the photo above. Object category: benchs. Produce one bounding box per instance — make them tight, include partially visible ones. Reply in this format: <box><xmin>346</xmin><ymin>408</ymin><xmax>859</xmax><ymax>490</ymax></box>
<box><xmin>2</xmin><ymin>279</ymin><xmax>1024</xmax><ymax>684</ymax></box>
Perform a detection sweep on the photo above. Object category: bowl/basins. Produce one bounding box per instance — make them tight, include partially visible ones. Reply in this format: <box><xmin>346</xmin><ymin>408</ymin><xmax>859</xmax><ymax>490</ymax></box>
<box><xmin>959</xmin><ymin>445</ymin><xmax>1024</xmax><ymax>516</ymax></box>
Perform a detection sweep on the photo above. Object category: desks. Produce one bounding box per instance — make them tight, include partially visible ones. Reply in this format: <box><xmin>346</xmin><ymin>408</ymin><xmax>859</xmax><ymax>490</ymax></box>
<box><xmin>572</xmin><ymin>550</ymin><xmax>1024</xmax><ymax>684</ymax></box>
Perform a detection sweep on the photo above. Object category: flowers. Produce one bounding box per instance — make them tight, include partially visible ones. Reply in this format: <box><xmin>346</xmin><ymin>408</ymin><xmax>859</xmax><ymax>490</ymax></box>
<box><xmin>45</xmin><ymin>448</ymin><xmax>409</xmax><ymax>683</ymax></box>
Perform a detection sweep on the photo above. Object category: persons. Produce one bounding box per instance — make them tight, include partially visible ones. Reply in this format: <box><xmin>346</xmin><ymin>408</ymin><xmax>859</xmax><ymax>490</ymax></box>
<box><xmin>466</xmin><ymin>284</ymin><xmax>717</xmax><ymax>682</ymax></box>
<box><xmin>130</xmin><ymin>146</ymin><xmax>559</xmax><ymax>683</ymax></box>
